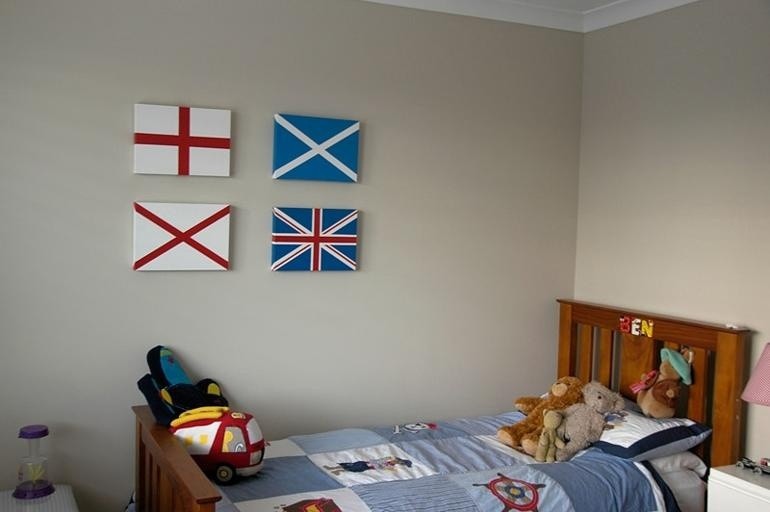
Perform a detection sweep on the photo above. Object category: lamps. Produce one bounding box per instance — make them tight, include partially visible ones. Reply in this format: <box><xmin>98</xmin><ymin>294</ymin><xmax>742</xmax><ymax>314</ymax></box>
<box><xmin>740</xmin><ymin>343</ymin><xmax>770</xmax><ymax>407</ymax></box>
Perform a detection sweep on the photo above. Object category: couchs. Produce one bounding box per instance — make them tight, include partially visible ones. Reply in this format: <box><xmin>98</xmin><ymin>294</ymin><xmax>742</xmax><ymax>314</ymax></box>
<box><xmin>130</xmin><ymin>298</ymin><xmax>752</xmax><ymax>511</ymax></box>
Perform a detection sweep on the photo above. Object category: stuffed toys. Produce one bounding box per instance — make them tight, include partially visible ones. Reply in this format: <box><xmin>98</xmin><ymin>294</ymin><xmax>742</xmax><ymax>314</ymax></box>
<box><xmin>496</xmin><ymin>376</ymin><xmax>626</xmax><ymax>462</ymax></box>
<box><xmin>629</xmin><ymin>347</ymin><xmax>695</xmax><ymax>420</ymax></box>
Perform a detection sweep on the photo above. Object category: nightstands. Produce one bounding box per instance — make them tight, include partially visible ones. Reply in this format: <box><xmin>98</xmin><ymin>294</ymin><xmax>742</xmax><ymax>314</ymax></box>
<box><xmin>706</xmin><ymin>460</ymin><xmax>770</xmax><ymax>512</ymax></box>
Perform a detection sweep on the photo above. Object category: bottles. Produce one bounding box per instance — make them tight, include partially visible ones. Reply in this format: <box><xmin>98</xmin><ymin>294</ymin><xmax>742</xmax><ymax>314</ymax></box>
<box><xmin>11</xmin><ymin>424</ymin><xmax>56</xmax><ymax>499</ymax></box>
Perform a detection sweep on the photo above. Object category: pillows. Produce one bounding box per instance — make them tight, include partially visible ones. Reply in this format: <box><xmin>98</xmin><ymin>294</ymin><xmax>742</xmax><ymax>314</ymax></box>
<box><xmin>591</xmin><ymin>408</ymin><xmax>712</xmax><ymax>463</ymax></box>
